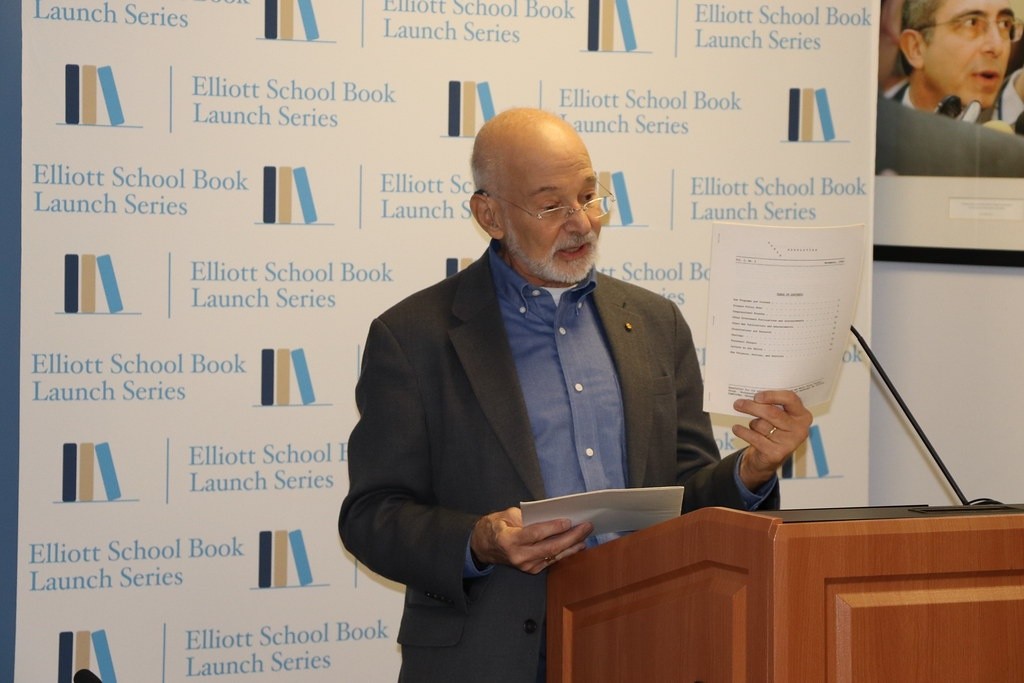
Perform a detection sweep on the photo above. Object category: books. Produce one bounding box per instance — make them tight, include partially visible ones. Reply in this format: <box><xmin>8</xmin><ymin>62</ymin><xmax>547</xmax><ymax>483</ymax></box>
<box><xmin>703</xmin><ymin>221</ymin><xmax>867</xmax><ymax>420</ymax></box>
<box><xmin>520</xmin><ymin>486</ymin><xmax>685</xmax><ymax>535</ymax></box>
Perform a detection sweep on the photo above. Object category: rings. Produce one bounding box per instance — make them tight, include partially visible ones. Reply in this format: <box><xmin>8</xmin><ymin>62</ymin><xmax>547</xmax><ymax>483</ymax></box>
<box><xmin>545</xmin><ymin>557</ymin><xmax>551</xmax><ymax>562</ymax></box>
<box><xmin>766</xmin><ymin>427</ymin><xmax>777</xmax><ymax>439</ymax></box>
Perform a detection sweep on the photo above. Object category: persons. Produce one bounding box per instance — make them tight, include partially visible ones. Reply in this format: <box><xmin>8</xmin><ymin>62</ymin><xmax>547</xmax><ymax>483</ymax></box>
<box><xmin>338</xmin><ymin>108</ymin><xmax>814</xmax><ymax>683</ymax></box>
<box><xmin>879</xmin><ymin>0</ymin><xmax>1024</xmax><ymax>135</ymax></box>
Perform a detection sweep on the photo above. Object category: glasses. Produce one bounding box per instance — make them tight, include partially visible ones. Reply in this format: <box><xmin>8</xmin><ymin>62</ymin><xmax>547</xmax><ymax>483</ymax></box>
<box><xmin>474</xmin><ymin>179</ymin><xmax>615</xmax><ymax>225</ymax></box>
<box><xmin>914</xmin><ymin>14</ymin><xmax>1024</xmax><ymax>42</ymax></box>
